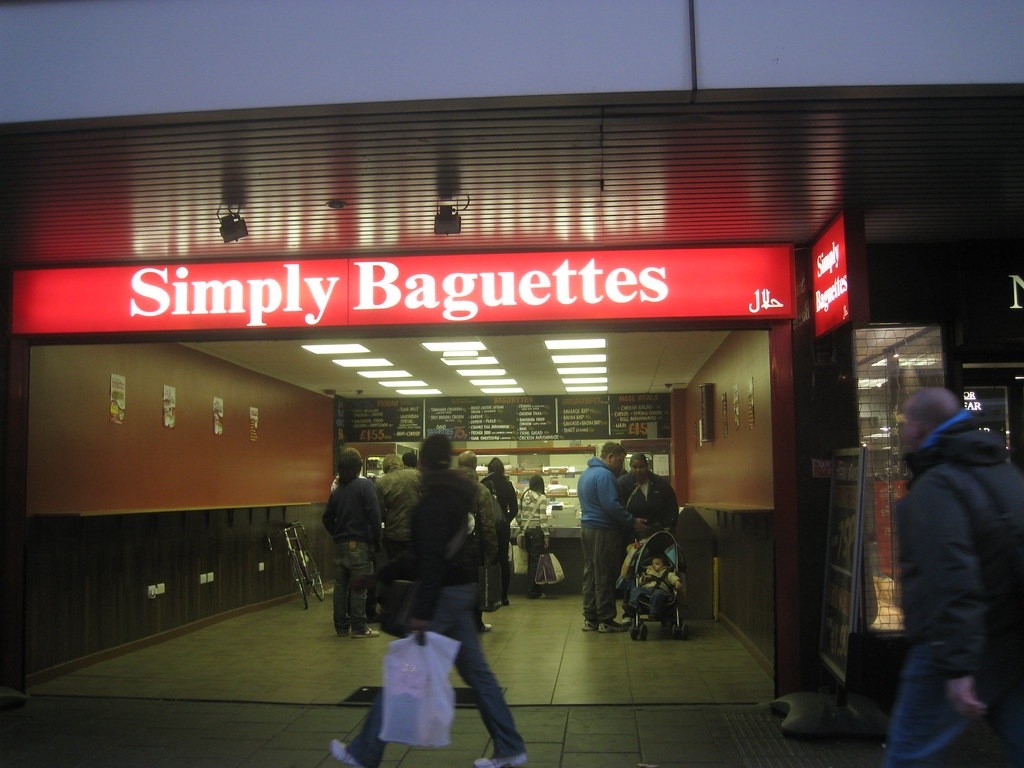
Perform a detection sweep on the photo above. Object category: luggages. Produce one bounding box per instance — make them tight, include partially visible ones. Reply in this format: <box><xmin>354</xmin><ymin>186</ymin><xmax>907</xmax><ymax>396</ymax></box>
<box><xmin>480</xmin><ymin>558</ymin><xmax>501</xmax><ymax>612</ymax></box>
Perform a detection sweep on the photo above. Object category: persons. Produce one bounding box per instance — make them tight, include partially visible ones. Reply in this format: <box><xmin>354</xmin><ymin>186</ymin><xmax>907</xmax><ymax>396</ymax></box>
<box><xmin>330</xmin><ymin>433</ymin><xmax>528</xmax><ymax>768</ymax></box>
<box><xmin>323</xmin><ymin>447</ymin><xmax>518</xmax><ymax>639</ymax></box>
<box><xmin>880</xmin><ymin>387</ymin><xmax>1024</xmax><ymax>768</ymax></box>
<box><xmin>577</xmin><ymin>442</ymin><xmax>683</xmax><ymax>633</ymax></box>
<box><xmin>516</xmin><ymin>474</ymin><xmax>550</xmax><ymax>600</ymax></box>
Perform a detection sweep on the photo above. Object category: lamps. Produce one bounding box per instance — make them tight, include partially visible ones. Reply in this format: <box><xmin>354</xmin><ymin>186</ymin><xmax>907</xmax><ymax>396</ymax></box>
<box><xmin>216</xmin><ymin>202</ymin><xmax>248</xmax><ymax>243</ymax></box>
<box><xmin>433</xmin><ymin>194</ymin><xmax>461</xmax><ymax>235</ymax></box>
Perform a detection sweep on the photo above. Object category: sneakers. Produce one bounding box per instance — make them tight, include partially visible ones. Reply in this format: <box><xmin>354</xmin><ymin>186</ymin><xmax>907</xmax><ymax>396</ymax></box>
<box><xmin>580</xmin><ymin>620</ymin><xmax>599</xmax><ymax>632</ymax></box>
<box><xmin>598</xmin><ymin>620</ymin><xmax>628</xmax><ymax>633</ymax></box>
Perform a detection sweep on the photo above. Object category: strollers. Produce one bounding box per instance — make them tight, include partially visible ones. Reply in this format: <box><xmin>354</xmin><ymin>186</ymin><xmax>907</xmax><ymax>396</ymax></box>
<box><xmin>615</xmin><ymin>529</ymin><xmax>690</xmax><ymax>641</ymax></box>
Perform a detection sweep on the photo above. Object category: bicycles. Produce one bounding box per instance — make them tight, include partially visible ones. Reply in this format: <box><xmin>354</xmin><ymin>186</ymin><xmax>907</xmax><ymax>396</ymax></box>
<box><xmin>264</xmin><ymin>520</ymin><xmax>325</xmax><ymax>610</ymax></box>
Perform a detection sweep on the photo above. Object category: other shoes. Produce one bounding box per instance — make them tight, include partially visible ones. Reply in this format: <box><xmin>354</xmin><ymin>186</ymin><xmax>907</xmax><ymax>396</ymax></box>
<box><xmin>329</xmin><ymin>739</ymin><xmax>365</xmax><ymax>768</ymax></box>
<box><xmin>479</xmin><ymin>624</ymin><xmax>491</xmax><ymax>632</ymax></box>
<box><xmin>350</xmin><ymin>627</ymin><xmax>379</xmax><ymax>638</ymax></box>
<box><xmin>648</xmin><ymin>613</ymin><xmax>660</xmax><ymax>621</ymax></box>
<box><xmin>475</xmin><ymin>751</ymin><xmax>528</xmax><ymax>768</ymax></box>
<box><xmin>622</xmin><ymin>604</ymin><xmax>636</xmax><ymax>618</ymax></box>
<box><xmin>336</xmin><ymin>629</ymin><xmax>350</xmax><ymax>637</ymax></box>
<box><xmin>526</xmin><ymin>590</ymin><xmax>544</xmax><ymax>599</ymax></box>
<box><xmin>622</xmin><ymin>611</ymin><xmax>631</xmax><ymax>622</ymax></box>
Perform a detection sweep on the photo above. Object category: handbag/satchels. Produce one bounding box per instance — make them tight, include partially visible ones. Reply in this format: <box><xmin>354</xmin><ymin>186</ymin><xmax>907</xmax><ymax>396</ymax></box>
<box><xmin>534</xmin><ymin>546</ymin><xmax>547</xmax><ymax>584</ymax></box>
<box><xmin>542</xmin><ymin>546</ymin><xmax>565</xmax><ymax>584</ymax></box>
<box><xmin>513</xmin><ymin>544</ymin><xmax>528</xmax><ymax>574</ymax></box>
<box><xmin>378</xmin><ymin>630</ymin><xmax>462</xmax><ymax>746</ymax></box>
<box><xmin>517</xmin><ymin>534</ymin><xmax>527</xmax><ymax>548</ymax></box>
